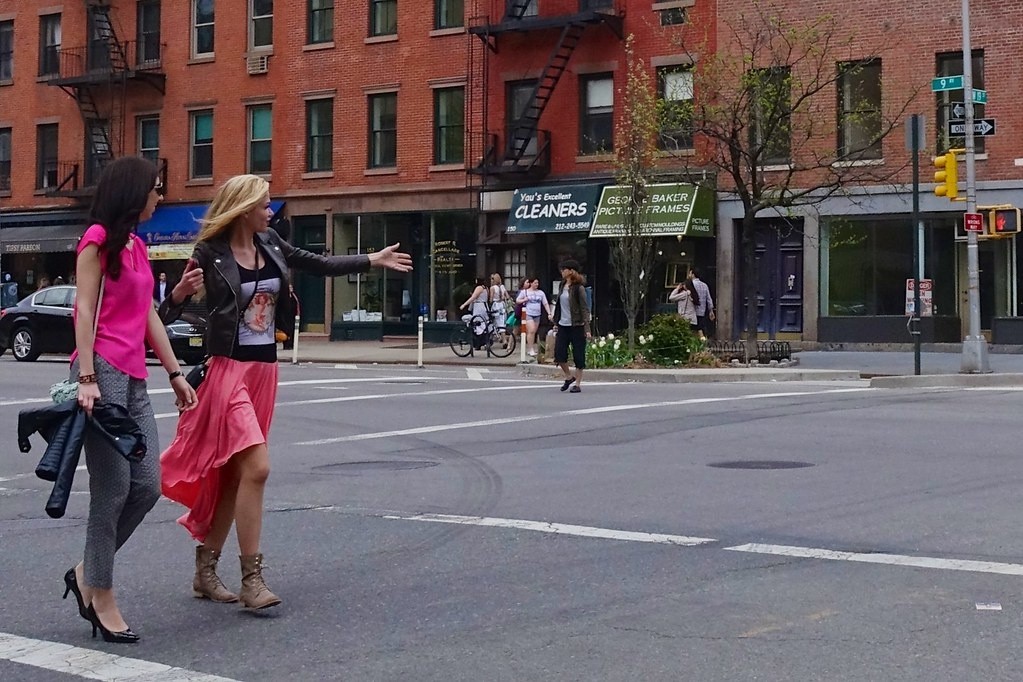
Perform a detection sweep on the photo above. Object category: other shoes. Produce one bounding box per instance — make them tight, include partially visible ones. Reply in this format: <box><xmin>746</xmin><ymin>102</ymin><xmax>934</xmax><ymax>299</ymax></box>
<box><xmin>527</xmin><ymin>351</ymin><xmax>538</xmax><ymax>356</ymax></box>
<box><xmin>560</xmin><ymin>376</ymin><xmax>576</xmax><ymax>391</ymax></box>
<box><xmin>475</xmin><ymin>346</ymin><xmax>481</xmax><ymax>350</ymax></box>
<box><xmin>503</xmin><ymin>341</ymin><xmax>507</xmax><ymax>349</ymax></box>
<box><xmin>570</xmin><ymin>385</ymin><xmax>582</xmax><ymax>393</ymax></box>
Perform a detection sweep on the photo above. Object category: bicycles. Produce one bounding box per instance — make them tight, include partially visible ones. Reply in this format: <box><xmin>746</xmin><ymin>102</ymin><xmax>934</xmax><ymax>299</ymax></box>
<box><xmin>449</xmin><ymin>308</ymin><xmax>517</xmax><ymax>358</ymax></box>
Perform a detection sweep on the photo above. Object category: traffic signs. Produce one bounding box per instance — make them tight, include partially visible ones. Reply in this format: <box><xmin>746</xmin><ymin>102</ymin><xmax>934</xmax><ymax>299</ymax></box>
<box><xmin>951</xmin><ymin>101</ymin><xmax>975</xmax><ymax>119</ymax></box>
<box><xmin>946</xmin><ymin>118</ymin><xmax>997</xmax><ymax>139</ymax></box>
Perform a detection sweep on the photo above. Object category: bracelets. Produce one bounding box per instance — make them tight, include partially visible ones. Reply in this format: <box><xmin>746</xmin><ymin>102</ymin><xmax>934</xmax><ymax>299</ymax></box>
<box><xmin>76</xmin><ymin>371</ymin><xmax>100</xmax><ymax>384</ymax></box>
<box><xmin>169</xmin><ymin>370</ymin><xmax>185</xmax><ymax>381</ymax></box>
<box><xmin>548</xmin><ymin>313</ymin><xmax>552</xmax><ymax>315</ymax></box>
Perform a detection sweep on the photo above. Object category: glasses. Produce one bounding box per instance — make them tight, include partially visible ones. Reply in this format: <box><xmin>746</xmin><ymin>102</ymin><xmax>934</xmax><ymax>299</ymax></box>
<box><xmin>153</xmin><ymin>181</ymin><xmax>164</xmax><ymax>196</ymax></box>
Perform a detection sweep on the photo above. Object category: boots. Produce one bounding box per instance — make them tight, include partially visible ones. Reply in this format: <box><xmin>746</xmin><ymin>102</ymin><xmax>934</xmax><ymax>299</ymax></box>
<box><xmin>237</xmin><ymin>554</ymin><xmax>282</xmax><ymax>610</ymax></box>
<box><xmin>190</xmin><ymin>545</ymin><xmax>240</xmax><ymax>602</ymax></box>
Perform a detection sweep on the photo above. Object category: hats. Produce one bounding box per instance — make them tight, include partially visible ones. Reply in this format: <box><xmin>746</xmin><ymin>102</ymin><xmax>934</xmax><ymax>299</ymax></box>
<box><xmin>558</xmin><ymin>260</ymin><xmax>579</xmax><ymax>272</ymax></box>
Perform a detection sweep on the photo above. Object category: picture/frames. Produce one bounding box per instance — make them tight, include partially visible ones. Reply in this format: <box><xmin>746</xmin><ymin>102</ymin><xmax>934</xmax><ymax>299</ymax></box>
<box><xmin>347</xmin><ymin>247</ymin><xmax>369</xmax><ymax>283</ymax></box>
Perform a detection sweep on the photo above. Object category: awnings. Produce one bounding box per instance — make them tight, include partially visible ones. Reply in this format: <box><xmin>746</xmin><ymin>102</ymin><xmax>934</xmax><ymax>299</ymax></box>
<box><xmin>505</xmin><ymin>177</ymin><xmax>718</xmax><ymax>243</ymax></box>
<box><xmin>0</xmin><ymin>198</ymin><xmax>286</xmax><ymax>258</ymax></box>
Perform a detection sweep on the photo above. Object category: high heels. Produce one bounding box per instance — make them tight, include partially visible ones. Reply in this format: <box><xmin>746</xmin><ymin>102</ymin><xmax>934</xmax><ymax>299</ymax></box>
<box><xmin>86</xmin><ymin>602</ymin><xmax>141</xmax><ymax>643</ymax></box>
<box><xmin>61</xmin><ymin>567</ymin><xmax>93</xmax><ymax>621</ymax></box>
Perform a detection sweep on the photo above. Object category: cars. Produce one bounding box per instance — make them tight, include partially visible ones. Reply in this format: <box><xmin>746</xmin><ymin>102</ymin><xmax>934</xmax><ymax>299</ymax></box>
<box><xmin>0</xmin><ymin>283</ymin><xmax>207</xmax><ymax>366</ymax></box>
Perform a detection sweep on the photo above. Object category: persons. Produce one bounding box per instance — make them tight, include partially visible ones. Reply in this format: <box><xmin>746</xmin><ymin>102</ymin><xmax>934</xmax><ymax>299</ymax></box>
<box><xmin>550</xmin><ymin>260</ymin><xmax>592</xmax><ymax>393</ymax></box>
<box><xmin>489</xmin><ymin>273</ymin><xmax>510</xmax><ymax>349</ymax></box>
<box><xmin>153</xmin><ymin>273</ymin><xmax>171</xmax><ymax>306</ymax></box>
<box><xmin>156</xmin><ymin>175</ymin><xmax>413</xmax><ymax>610</ymax></box>
<box><xmin>669</xmin><ymin>266</ymin><xmax>715</xmax><ymax>337</ymax></box>
<box><xmin>459</xmin><ymin>271</ymin><xmax>490</xmax><ymax>350</ymax></box>
<box><xmin>514</xmin><ymin>276</ymin><xmax>553</xmax><ymax>357</ymax></box>
<box><xmin>62</xmin><ymin>156</ymin><xmax>198</xmax><ymax>644</ymax></box>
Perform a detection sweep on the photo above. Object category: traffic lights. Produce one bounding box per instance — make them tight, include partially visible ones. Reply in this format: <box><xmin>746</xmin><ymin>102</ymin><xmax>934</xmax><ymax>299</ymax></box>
<box><xmin>934</xmin><ymin>148</ymin><xmax>966</xmax><ymax>202</ymax></box>
<box><xmin>991</xmin><ymin>207</ymin><xmax>1021</xmax><ymax>233</ymax></box>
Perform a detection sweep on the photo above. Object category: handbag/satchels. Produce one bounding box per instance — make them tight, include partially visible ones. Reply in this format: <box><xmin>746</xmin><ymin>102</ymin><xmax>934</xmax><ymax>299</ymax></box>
<box><xmin>514</xmin><ymin>302</ymin><xmax>523</xmax><ymax>319</ymax></box>
<box><xmin>49</xmin><ymin>379</ymin><xmax>79</xmax><ymax>404</ymax></box>
<box><xmin>184</xmin><ymin>362</ymin><xmax>210</xmax><ymax>387</ymax></box>
<box><xmin>504</xmin><ymin>299</ymin><xmax>514</xmax><ymax>315</ymax></box>
